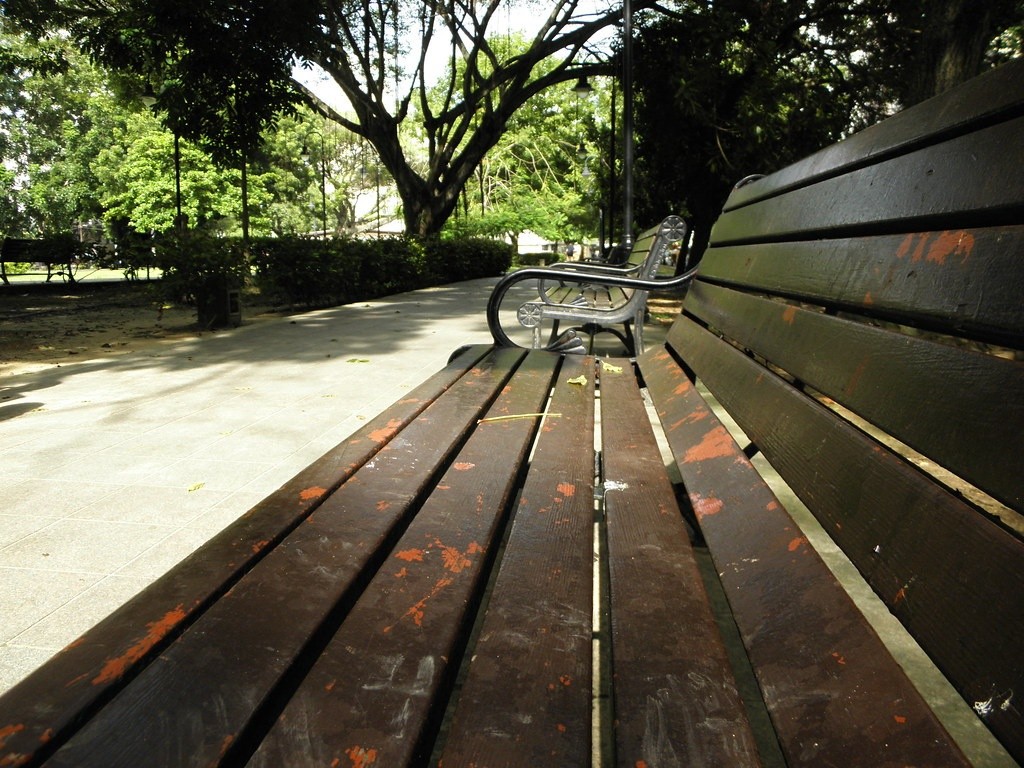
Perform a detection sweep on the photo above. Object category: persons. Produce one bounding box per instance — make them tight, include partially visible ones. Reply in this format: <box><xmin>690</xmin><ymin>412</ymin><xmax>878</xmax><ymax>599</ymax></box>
<box><xmin>566</xmin><ymin>241</ymin><xmax>582</xmax><ymax>261</ymax></box>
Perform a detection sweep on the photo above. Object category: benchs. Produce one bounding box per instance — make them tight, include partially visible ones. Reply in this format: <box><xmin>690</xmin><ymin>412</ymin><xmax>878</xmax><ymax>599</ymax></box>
<box><xmin>575</xmin><ymin>247</ymin><xmax>615</xmax><ymax>285</ymax></box>
<box><xmin>0</xmin><ymin>236</ymin><xmax>80</xmax><ymax>286</ymax></box>
<box><xmin>515</xmin><ymin>214</ymin><xmax>687</xmax><ymax>357</ymax></box>
<box><xmin>1</xmin><ymin>59</ymin><xmax>1024</xmax><ymax>768</ymax></box>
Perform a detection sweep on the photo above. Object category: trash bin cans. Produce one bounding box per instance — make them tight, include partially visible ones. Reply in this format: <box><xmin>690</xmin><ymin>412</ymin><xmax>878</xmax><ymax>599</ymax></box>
<box><xmin>193</xmin><ymin>271</ymin><xmax>242</xmax><ymax>332</ymax></box>
